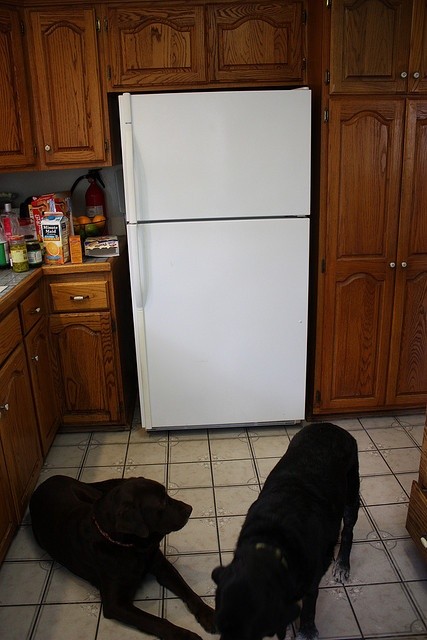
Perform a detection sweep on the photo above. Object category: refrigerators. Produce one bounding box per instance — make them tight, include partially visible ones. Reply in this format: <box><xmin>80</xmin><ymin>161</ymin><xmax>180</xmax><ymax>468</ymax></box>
<box><xmin>119</xmin><ymin>89</ymin><xmax>310</xmax><ymax>432</ymax></box>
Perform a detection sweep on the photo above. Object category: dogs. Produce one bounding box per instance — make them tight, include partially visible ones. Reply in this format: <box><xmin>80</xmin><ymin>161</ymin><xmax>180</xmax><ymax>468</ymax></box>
<box><xmin>208</xmin><ymin>421</ymin><xmax>363</xmax><ymax>639</ymax></box>
<box><xmin>26</xmin><ymin>472</ymin><xmax>222</xmax><ymax>640</ymax></box>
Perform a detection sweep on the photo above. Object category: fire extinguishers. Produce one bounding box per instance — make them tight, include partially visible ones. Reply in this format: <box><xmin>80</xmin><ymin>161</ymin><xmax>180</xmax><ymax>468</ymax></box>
<box><xmin>71</xmin><ymin>168</ymin><xmax>108</xmax><ymax>236</ymax></box>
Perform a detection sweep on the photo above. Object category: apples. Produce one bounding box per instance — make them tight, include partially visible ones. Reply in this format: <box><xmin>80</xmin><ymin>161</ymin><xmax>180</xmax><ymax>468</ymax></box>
<box><xmin>85</xmin><ymin>224</ymin><xmax>99</xmax><ymax>235</ymax></box>
<box><xmin>78</xmin><ymin>231</ymin><xmax>87</xmax><ymax>241</ymax></box>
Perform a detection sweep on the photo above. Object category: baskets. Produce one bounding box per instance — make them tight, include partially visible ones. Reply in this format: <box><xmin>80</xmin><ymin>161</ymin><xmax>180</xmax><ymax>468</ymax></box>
<box><xmin>72</xmin><ymin>217</ymin><xmax>106</xmax><ymax>247</ymax></box>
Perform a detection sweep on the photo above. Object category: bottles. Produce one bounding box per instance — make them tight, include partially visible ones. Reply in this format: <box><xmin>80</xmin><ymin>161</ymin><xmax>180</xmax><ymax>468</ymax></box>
<box><xmin>1</xmin><ymin>204</ymin><xmax>19</xmax><ymax>238</ymax></box>
<box><xmin>0</xmin><ymin>222</ymin><xmax>10</xmax><ymax>268</ymax></box>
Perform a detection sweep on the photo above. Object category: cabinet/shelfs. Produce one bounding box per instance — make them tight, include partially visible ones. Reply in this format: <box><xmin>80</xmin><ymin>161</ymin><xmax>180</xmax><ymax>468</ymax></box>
<box><xmin>103</xmin><ymin>1</ymin><xmax>206</xmax><ymax>92</ymax></box>
<box><xmin>406</xmin><ymin>418</ymin><xmax>427</xmax><ymax>560</ymax></box>
<box><xmin>0</xmin><ymin>308</ymin><xmax>45</xmax><ymax>524</ymax></box>
<box><xmin>0</xmin><ymin>444</ymin><xmax>20</xmax><ymax>567</ymax></box>
<box><xmin>42</xmin><ymin>256</ymin><xmax>131</xmax><ymax>434</ymax></box>
<box><xmin>312</xmin><ymin>100</ymin><xmax>425</xmax><ymax>416</ymax></box>
<box><xmin>305</xmin><ymin>0</ymin><xmax>426</xmax><ymax>99</ymax></box>
<box><xmin>0</xmin><ymin>0</ymin><xmax>37</xmax><ymax>174</ymax></box>
<box><xmin>22</xmin><ymin>1</ymin><xmax>113</xmax><ymax>172</ymax></box>
<box><xmin>18</xmin><ymin>282</ymin><xmax>62</xmax><ymax>461</ymax></box>
<box><xmin>206</xmin><ymin>2</ymin><xmax>306</xmax><ymax>87</ymax></box>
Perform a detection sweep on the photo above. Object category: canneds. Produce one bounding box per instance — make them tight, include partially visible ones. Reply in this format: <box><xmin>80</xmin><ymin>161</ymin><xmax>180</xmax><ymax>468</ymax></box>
<box><xmin>9</xmin><ymin>235</ymin><xmax>29</xmax><ymax>273</ymax></box>
<box><xmin>27</xmin><ymin>240</ymin><xmax>42</xmax><ymax>268</ymax></box>
